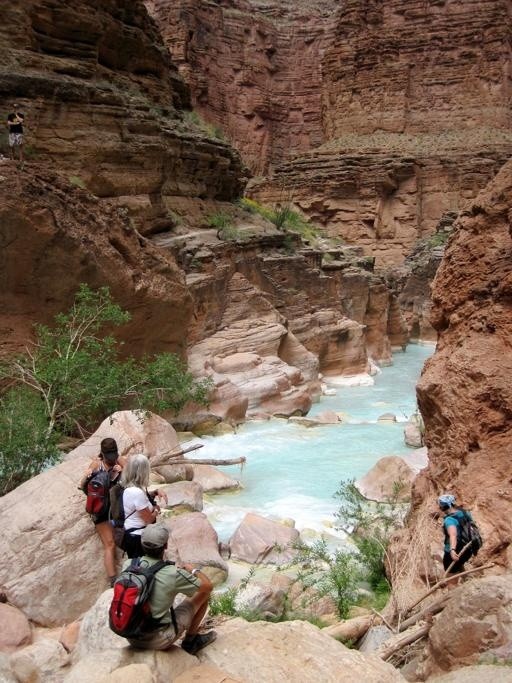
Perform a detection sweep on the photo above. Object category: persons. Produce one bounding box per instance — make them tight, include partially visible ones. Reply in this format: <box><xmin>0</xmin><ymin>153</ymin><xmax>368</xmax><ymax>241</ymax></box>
<box><xmin>106</xmin><ymin>523</ymin><xmax>219</xmax><ymax>655</ymax></box>
<box><xmin>104</xmin><ymin>454</ymin><xmax>171</xmax><ymax>563</ymax></box>
<box><xmin>437</xmin><ymin>494</ymin><xmax>475</xmax><ymax>586</ymax></box>
<box><xmin>7</xmin><ymin>102</ymin><xmax>26</xmax><ymax>161</ymax></box>
<box><xmin>76</xmin><ymin>437</ymin><xmax>131</xmax><ymax>589</ymax></box>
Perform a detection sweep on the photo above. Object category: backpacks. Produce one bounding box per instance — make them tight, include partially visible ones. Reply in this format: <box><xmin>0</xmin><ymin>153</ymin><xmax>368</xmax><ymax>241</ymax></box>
<box><xmin>105</xmin><ymin>556</ymin><xmax>174</xmax><ymax>638</ymax></box>
<box><xmin>107</xmin><ymin>484</ymin><xmax>126</xmax><ymax>548</ymax></box>
<box><xmin>448</xmin><ymin>510</ymin><xmax>483</xmax><ymax>553</ymax></box>
<box><xmin>84</xmin><ymin>470</ymin><xmax>112</xmax><ymax>514</ymax></box>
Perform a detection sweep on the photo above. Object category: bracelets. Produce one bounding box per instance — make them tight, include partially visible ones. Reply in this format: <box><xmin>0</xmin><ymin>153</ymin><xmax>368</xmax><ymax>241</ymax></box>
<box><xmin>191</xmin><ymin>568</ymin><xmax>200</xmax><ymax>575</ymax></box>
<box><xmin>449</xmin><ymin>548</ymin><xmax>456</xmax><ymax>552</ymax></box>
<box><xmin>154</xmin><ymin>509</ymin><xmax>159</xmax><ymax>516</ymax></box>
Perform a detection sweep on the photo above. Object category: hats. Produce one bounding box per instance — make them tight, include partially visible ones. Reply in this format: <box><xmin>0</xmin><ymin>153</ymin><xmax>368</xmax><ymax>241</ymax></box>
<box><xmin>140</xmin><ymin>523</ymin><xmax>172</xmax><ymax>550</ymax></box>
<box><xmin>98</xmin><ymin>438</ymin><xmax>118</xmax><ymax>459</ymax></box>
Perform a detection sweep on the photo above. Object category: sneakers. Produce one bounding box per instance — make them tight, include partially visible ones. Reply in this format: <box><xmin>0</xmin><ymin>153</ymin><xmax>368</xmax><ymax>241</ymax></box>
<box><xmin>182</xmin><ymin>629</ymin><xmax>217</xmax><ymax>657</ymax></box>
